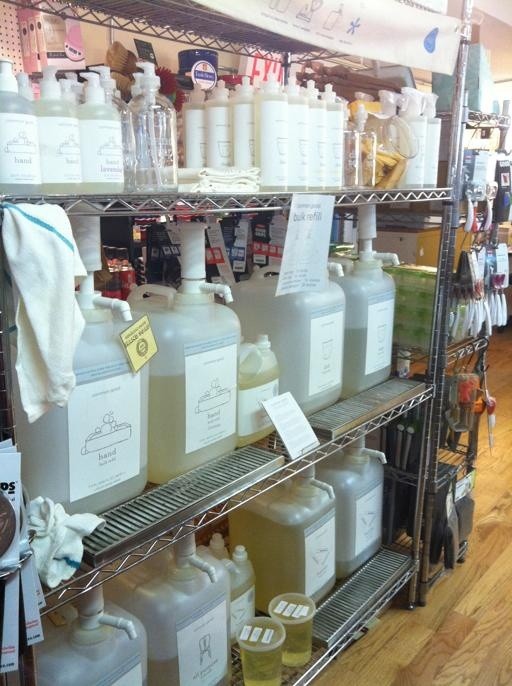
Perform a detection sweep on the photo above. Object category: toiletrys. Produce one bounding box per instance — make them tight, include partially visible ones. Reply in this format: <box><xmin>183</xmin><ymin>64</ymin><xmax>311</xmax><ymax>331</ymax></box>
<box><xmin>0</xmin><ymin>58</ymin><xmax>442</xmax><ymax>199</ymax></box>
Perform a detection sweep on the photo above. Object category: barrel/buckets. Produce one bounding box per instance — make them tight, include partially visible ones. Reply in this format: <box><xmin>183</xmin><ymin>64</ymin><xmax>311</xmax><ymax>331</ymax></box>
<box><xmin>328</xmin><ymin>256</ymin><xmax>397</xmax><ymax>401</ymax></box>
<box><xmin>196</xmin><ymin>532</ymin><xmax>258</xmax><ymax>648</ymax></box>
<box><xmin>306</xmin><ymin>442</ymin><xmax>385</xmax><ymax>580</ymax></box>
<box><xmin>102</xmin><ymin>537</ymin><xmax>232</xmax><ymax>686</ymax></box>
<box><xmin>227</xmin><ymin>472</ymin><xmax>337</xmax><ymax>616</ymax></box>
<box><xmin>226</xmin><ymin>264</ymin><xmax>346</xmax><ymax>418</ymax></box>
<box><xmin>236</xmin><ymin>333</ymin><xmax>280</xmax><ymax>449</ymax></box>
<box><xmin>126</xmin><ymin>283</ymin><xmax>241</xmax><ymax>485</ymax></box>
<box><xmin>7</xmin><ymin>308</ymin><xmax>149</xmax><ymax>516</ymax></box>
<box><xmin>33</xmin><ymin>584</ymin><xmax>149</xmax><ymax>686</ymax></box>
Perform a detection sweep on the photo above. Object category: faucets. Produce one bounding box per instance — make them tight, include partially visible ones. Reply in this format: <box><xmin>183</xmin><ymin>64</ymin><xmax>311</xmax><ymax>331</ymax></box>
<box><xmin>326</xmin><ymin>261</ymin><xmax>344</xmax><ymax>278</ymax></box>
<box><xmin>347</xmin><ymin>424</ymin><xmax>388</xmax><ymax>465</ymax></box>
<box><xmin>75</xmin><ymin>569</ymin><xmax>138</xmax><ymax>640</ymax></box>
<box><xmin>64</xmin><ymin>201</ymin><xmax>133</xmax><ymax>322</ymax></box>
<box><xmin>177</xmin><ymin>222</ymin><xmax>233</xmax><ymax>304</ymax></box>
<box><xmin>172</xmin><ymin>519</ymin><xmax>217</xmax><ymax>583</ymax></box>
<box><xmin>357</xmin><ymin>204</ymin><xmax>401</xmax><ymax>267</ymax></box>
<box><xmin>296</xmin><ymin>453</ymin><xmax>335</xmax><ymax>499</ymax></box>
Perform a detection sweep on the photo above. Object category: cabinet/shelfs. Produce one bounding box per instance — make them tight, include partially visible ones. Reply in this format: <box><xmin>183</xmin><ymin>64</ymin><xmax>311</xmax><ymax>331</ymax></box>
<box><xmin>0</xmin><ymin>0</ymin><xmax>512</xmax><ymax>686</ymax></box>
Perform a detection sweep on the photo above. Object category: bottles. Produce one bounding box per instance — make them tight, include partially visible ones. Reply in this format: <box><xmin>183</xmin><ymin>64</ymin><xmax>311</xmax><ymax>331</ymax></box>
<box><xmin>183</xmin><ymin>72</ymin><xmax>348</xmax><ymax>188</ymax></box>
<box><xmin>355</xmin><ymin>81</ymin><xmax>441</xmax><ymax>192</ymax></box>
<box><xmin>99</xmin><ymin>245</ymin><xmax>140</xmax><ymax>299</ymax></box>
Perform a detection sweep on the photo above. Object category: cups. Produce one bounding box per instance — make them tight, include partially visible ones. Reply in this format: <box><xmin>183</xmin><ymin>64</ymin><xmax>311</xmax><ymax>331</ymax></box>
<box><xmin>237</xmin><ymin>592</ymin><xmax>319</xmax><ymax>686</ymax></box>
